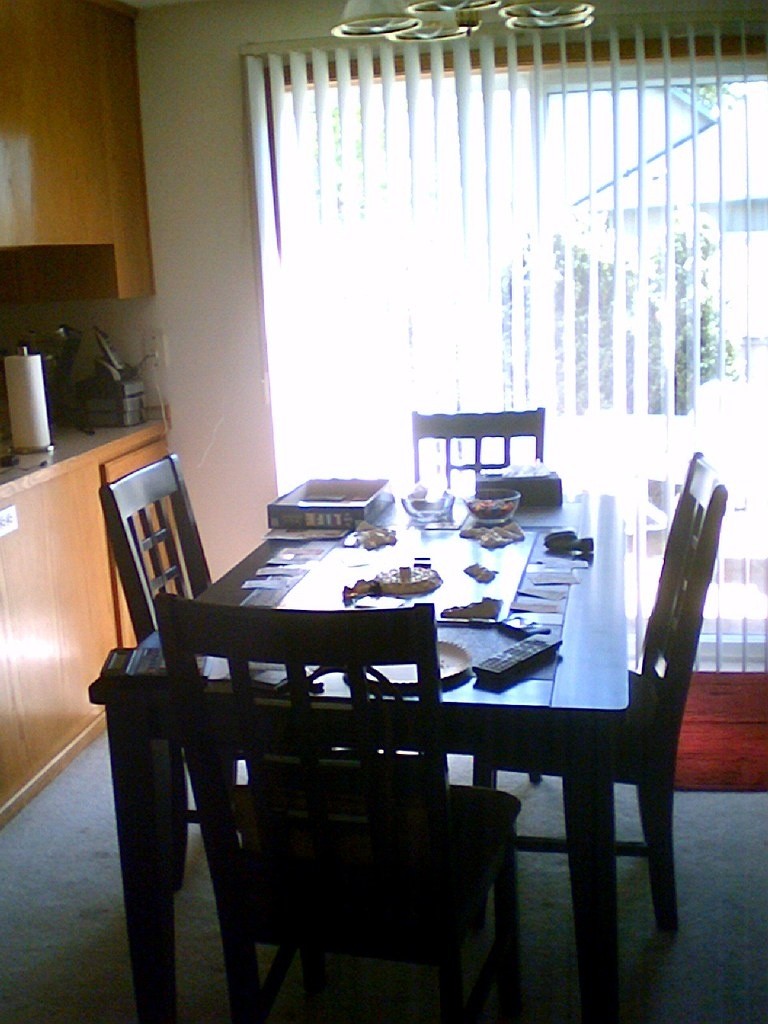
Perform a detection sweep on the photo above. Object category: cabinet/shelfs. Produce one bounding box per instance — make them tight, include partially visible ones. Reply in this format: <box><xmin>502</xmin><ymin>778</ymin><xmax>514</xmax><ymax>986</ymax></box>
<box><xmin>0</xmin><ymin>436</ymin><xmax>193</xmax><ymax>830</ymax></box>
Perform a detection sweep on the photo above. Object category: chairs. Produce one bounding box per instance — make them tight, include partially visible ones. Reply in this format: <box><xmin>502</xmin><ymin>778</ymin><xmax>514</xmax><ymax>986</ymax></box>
<box><xmin>90</xmin><ymin>409</ymin><xmax>726</xmax><ymax>1024</ymax></box>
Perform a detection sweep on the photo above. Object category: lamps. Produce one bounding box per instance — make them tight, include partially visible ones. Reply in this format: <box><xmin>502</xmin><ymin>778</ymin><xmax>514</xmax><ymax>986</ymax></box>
<box><xmin>330</xmin><ymin>0</ymin><xmax>604</xmax><ymax>45</ymax></box>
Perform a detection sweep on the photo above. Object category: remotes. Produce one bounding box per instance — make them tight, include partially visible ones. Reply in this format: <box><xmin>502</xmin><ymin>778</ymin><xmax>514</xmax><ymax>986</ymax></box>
<box><xmin>470</xmin><ymin>634</ymin><xmax>563</xmax><ymax>682</ymax></box>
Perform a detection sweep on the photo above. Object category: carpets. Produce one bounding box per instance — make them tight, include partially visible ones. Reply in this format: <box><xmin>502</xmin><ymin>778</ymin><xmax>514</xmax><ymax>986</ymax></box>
<box><xmin>671</xmin><ymin>673</ymin><xmax>767</xmax><ymax>791</ymax></box>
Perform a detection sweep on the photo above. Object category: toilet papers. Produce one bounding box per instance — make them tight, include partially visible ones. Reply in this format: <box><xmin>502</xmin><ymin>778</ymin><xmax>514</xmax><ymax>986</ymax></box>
<box><xmin>3</xmin><ymin>352</ymin><xmax>51</xmax><ymax>449</ymax></box>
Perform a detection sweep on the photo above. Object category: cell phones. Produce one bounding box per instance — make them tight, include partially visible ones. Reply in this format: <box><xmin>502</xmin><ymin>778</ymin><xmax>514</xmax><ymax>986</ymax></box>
<box><xmin>502</xmin><ymin>618</ymin><xmax>551</xmax><ymax>637</ymax></box>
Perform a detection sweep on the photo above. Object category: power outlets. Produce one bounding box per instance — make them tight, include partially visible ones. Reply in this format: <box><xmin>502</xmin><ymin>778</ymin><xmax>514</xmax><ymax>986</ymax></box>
<box><xmin>143</xmin><ymin>328</ymin><xmax>171</xmax><ymax>370</ymax></box>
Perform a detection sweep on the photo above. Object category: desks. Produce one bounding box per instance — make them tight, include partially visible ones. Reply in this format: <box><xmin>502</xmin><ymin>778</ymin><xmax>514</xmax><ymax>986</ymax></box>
<box><xmin>89</xmin><ymin>476</ymin><xmax>628</xmax><ymax>1024</ymax></box>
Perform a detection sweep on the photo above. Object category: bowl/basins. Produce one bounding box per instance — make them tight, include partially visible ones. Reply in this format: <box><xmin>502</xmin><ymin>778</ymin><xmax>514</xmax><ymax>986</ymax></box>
<box><xmin>400</xmin><ymin>497</ymin><xmax>457</xmax><ymax>518</ymax></box>
<box><xmin>464</xmin><ymin>489</ymin><xmax>522</xmax><ymax>523</ymax></box>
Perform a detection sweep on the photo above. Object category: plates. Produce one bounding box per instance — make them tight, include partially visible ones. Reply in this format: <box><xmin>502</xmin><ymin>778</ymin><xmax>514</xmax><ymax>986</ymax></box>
<box><xmin>366</xmin><ymin>641</ymin><xmax>472</xmax><ymax>683</ymax></box>
